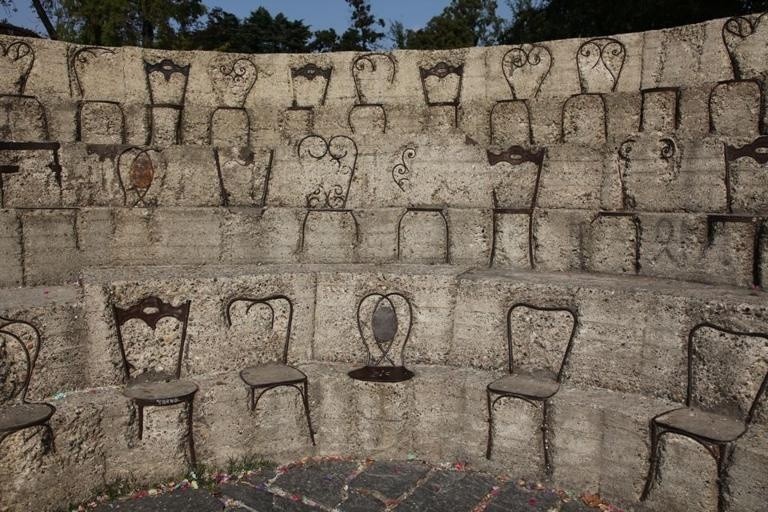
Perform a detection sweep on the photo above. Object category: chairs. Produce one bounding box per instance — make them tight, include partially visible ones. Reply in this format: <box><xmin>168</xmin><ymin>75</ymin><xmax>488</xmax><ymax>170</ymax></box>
<box><xmin>0</xmin><ymin>41</ymin><xmax>767</xmax><ymax>512</ymax></box>
